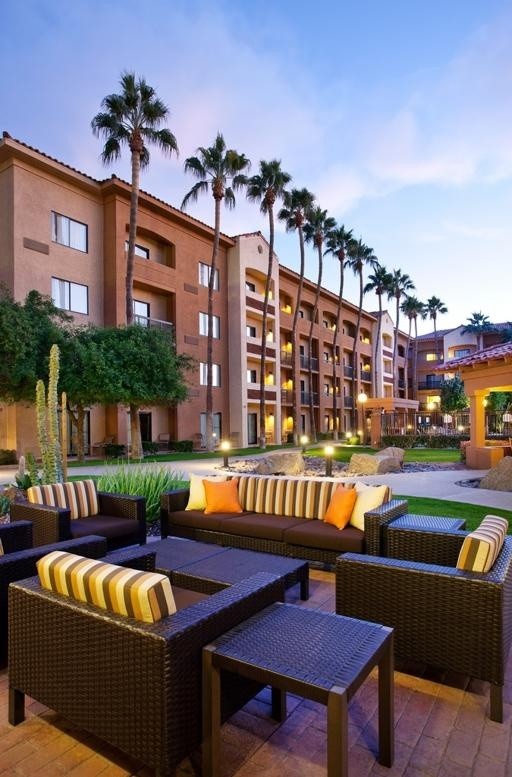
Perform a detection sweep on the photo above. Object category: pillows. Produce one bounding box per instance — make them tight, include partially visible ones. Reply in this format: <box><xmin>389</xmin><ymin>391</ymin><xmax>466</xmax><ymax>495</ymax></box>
<box><xmin>317</xmin><ymin>481</ymin><xmax>356</xmax><ymax>529</ymax></box>
<box><xmin>181</xmin><ymin>471</ymin><xmax>227</xmax><ymax>512</ymax></box>
<box><xmin>350</xmin><ymin>479</ymin><xmax>389</xmax><ymax>533</ymax></box>
<box><xmin>201</xmin><ymin>475</ymin><xmax>243</xmax><ymax>514</ymax></box>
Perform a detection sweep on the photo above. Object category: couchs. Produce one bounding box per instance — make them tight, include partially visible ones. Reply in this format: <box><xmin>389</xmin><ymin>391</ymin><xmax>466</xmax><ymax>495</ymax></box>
<box><xmin>157</xmin><ymin>469</ymin><xmax>407</xmax><ymax>572</ymax></box>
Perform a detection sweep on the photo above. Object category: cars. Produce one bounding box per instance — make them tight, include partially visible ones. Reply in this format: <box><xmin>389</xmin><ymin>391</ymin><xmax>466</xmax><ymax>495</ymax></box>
<box><xmin>386</xmin><ymin>422</ymin><xmax>463</xmax><ymax>436</ymax></box>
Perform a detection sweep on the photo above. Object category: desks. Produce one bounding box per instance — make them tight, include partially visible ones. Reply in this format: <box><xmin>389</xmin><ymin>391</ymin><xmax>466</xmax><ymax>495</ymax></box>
<box><xmin>385</xmin><ymin>514</ymin><xmax>466</xmax><ymax>533</ymax></box>
<box><xmin>199</xmin><ymin>597</ymin><xmax>395</xmax><ymax>777</ymax></box>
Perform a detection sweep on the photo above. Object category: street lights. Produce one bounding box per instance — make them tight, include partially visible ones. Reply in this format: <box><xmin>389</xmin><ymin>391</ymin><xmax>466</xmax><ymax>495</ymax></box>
<box><xmin>357</xmin><ymin>393</ymin><xmax>369</xmax><ymax>445</ymax></box>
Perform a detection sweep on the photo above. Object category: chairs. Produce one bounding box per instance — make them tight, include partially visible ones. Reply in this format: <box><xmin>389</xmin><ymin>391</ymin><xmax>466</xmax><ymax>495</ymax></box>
<box><xmin>8</xmin><ymin>479</ymin><xmax>149</xmax><ymax>554</ymax></box>
<box><xmin>334</xmin><ymin>511</ymin><xmax>512</xmax><ymax>724</ymax></box>
<box><xmin>3</xmin><ymin>534</ymin><xmax>289</xmax><ymax>775</ymax></box>
<box><xmin>0</xmin><ymin>516</ymin><xmax>109</xmax><ymax>667</ymax></box>
